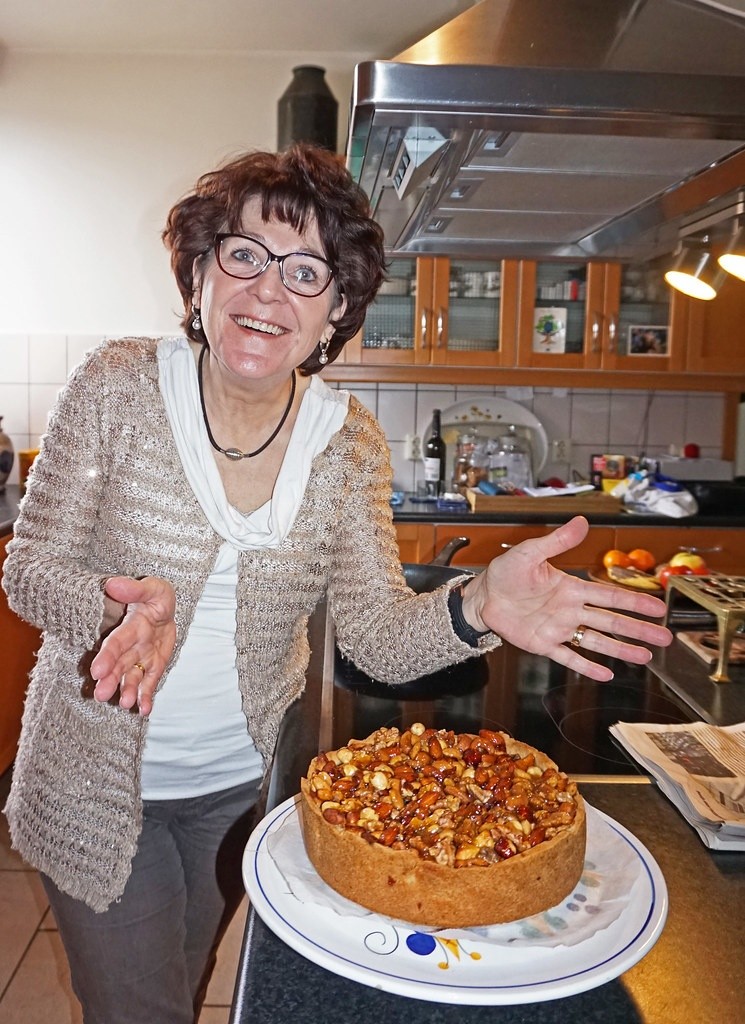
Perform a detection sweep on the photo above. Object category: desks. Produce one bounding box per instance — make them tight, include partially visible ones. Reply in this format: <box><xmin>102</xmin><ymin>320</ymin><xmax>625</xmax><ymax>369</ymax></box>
<box><xmin>230</xmin><ymin>772</ymin><xmax>745</xmax><ymax>1024</ymax></box>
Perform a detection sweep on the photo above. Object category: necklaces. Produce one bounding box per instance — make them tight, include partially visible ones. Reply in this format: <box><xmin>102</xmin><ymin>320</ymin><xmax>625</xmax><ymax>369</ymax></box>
<box><xmin>198</xmin><ymin>345</ymin><xmax>296</xmax><ymax>460</ymax></box>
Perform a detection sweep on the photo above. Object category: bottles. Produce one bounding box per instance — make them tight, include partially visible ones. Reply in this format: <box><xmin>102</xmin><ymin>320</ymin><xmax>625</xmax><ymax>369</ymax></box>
<box><xmin>425</xmin><ymin>409</ymin><xmax>445</xmax><ymax>496</ymax></box>
<box><xmin>0</xmin><ymin>416</ymin><xmax>14</xmax><ymax>493</ymax></box>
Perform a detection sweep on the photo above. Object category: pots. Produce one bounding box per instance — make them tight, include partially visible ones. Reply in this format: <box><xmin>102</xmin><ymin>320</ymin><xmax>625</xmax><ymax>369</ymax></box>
<box><xmin>334</xmin><ymin>536</ymin><xmax>489</xmax><ymax>700</ymax></box>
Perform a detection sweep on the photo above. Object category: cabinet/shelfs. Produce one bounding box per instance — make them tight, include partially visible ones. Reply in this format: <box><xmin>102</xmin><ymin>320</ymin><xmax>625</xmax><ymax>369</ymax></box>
<box><xmin>319</xmin><ymin>249</ymin><xmax>745</xmax><ymax>403</ymax></box>
<box><xmin>324</xmin><ymin>513</ymin><xmax>745</xmax><ymax>775</ymax></box>
<box><xmin>0</xmin><ymin>478</ymin><xmax>41</xmax><ymax>778</ymax></box>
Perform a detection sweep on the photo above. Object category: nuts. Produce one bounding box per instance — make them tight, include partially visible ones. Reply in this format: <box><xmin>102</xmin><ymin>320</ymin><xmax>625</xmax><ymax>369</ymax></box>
<box><xmin>312</xmin><ymin>724</ymin><xmax>579</xmax><ymax>871</ymax></box>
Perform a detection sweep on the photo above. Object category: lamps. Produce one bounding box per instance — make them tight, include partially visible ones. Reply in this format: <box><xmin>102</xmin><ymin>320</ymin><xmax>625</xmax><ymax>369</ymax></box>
<box><xmin>664</xmin><ymin>184</ymin><xmax>745</xmax><ymax>300</ymax></box>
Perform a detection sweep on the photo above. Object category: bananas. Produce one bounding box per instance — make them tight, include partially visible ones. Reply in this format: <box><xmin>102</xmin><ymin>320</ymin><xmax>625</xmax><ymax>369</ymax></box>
<box><xmin>607</xmin><ymin>565</ymin><xmax>663</xmax><ymax>589</ymax></box>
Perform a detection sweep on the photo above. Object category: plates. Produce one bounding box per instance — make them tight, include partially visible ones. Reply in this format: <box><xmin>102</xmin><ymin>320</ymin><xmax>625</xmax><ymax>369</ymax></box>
<box><xmin>587</xmin><ymin>567</ymin><xmax>664</xmax><ymax>595</ymax></box>
<box><xmin>241</xmin><ymin>788</ymin><xmax>668</xmax><ymax>1005</ymax></box>
<box><xmin>421</xmin><ymin>396</ymin><xmax>548</xmax><ymax>475</ymax></box>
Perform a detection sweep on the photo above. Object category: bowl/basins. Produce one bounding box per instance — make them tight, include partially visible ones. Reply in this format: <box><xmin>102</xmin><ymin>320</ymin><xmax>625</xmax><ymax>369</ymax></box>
<box><xmin>602</xmin><ymin>479</ymin><xmax>627</xmax><ymax>494</ymax></box>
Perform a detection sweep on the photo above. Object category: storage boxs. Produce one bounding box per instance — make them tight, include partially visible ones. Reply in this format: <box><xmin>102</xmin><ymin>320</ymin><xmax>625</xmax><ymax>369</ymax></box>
<box><xmin>655</xmin><ymin>458</ymin><xmax>733</xmax><ymax>480</ymax></box>
<box><xmin>590</xmin><ymin>454</ymin><xmax>633</xmax><ymax>492</ymax></box>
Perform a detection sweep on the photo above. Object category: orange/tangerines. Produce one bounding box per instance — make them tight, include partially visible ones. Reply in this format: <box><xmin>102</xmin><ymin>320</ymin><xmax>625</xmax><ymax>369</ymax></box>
<box><xmin>605</xmin><ymin>548</ymin><xmax>655</xmax><ymax>571</ymax></box>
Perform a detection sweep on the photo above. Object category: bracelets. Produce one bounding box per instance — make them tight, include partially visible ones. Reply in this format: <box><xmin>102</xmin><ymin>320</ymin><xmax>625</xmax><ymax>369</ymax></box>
<box><xmin>448</xmin><ymin>577</ymin><xmax>493</xmax><ymax>648</ymax></box>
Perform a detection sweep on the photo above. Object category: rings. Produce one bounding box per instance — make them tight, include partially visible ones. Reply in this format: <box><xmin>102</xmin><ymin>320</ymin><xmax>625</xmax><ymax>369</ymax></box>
<box><xmin>135</xmin><ymin>664</ymin><xmax>145</xmax><ymax>676</ymax></box>
<box><xmin>572</xmin><ymin>626</ymin><xmax>586</xmax><ymax>646</ymax></box>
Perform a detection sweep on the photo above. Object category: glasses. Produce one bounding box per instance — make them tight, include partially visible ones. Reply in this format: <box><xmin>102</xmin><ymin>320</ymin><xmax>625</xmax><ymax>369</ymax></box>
<box><xmin>203</xmin><ymin>233</ymin><xmax>343</xmax><ymax>297</ymax></box>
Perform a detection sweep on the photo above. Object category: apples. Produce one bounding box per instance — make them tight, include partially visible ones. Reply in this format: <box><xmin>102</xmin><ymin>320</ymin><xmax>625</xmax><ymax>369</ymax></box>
<box><xmin>658</xmin><ymin>552</ymin><xmax>717</xmax><ymax>592</ymax></box>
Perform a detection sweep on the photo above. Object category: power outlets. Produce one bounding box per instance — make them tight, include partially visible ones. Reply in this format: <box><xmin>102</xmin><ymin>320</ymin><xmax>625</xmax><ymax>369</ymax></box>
<box><xmin>553</xmin><ymin>440</ymin><xmax>571</xmax><ymax>464</ymax></box>
<box><xmin>406</xmin><ymin>434</ymin><xmax>423</xmax><ymax>460</ymax></box>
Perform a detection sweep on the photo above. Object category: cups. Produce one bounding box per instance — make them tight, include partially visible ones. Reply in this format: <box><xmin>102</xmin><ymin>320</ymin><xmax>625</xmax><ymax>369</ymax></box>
<box><xmin>18</xmin><ymin>447</ymin><xmax>41</xmax><ymax>501</ymax></box>
<box><xmin>543</xmin><ymin>279</ymin><xmax>577</xmax><ymax>300</ymax></box>
<box><xmin>410</xmin><ymin>271</ymin><xmax>501</xmax><ymax>298</ymax></box>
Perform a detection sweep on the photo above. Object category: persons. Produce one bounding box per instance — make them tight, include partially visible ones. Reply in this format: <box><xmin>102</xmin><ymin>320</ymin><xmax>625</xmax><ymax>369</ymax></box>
<box><xmin>0</xmin><ymin>144</ymin><xmax>672</xmax><ymax>1024</ymax></box>
<box><xmin>631</xmin><ymin>330</ymin><xmax>666</xmax><ymax>354</ymax></box>
